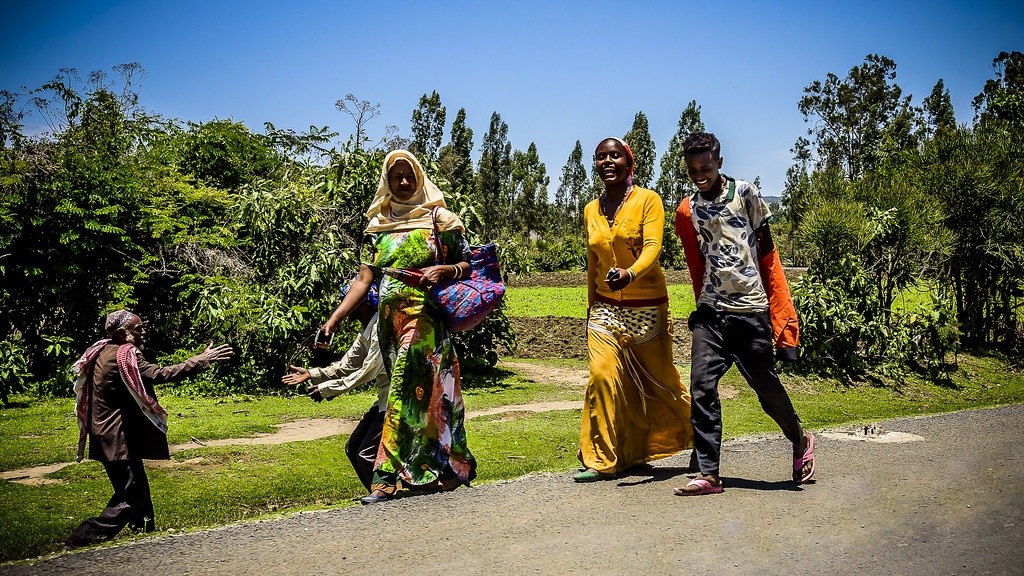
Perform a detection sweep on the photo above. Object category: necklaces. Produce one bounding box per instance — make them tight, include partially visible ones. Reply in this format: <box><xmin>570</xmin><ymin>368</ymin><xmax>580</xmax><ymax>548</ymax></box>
<box><xmin>602</xmin><ymin>185</ymin><xmax>631</xmax><ymax>226</ymax></box>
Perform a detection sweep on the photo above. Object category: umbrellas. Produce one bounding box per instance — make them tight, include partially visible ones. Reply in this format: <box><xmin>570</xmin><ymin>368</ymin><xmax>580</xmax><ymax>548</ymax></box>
<box><xmin>361</xmin><ymin>261</ymin><xmax>425</xmax><ymax>287</ymax></box>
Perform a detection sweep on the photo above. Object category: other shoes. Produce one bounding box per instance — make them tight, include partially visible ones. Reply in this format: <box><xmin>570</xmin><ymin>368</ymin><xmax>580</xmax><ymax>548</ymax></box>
<box><xmin>574</xmin><ymin>468</ymin><xmax>617</xmax><ymax>482</ymax></box>
<box><xmin>689</xmin><ymin>448</ymin><xmax>701</xmax><ymax>473</ymax></box>
<box><xmin>442</xmin><ymin>477</ymin><xmax>470</xmax><ymax>491</ymax></box>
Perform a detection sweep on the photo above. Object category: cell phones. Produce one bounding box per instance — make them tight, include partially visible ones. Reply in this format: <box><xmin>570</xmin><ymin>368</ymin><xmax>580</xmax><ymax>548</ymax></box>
<box><xmin>315</xmin><ymin>330</ymin><xmax>335</xmax><ymax>346</ymax></box>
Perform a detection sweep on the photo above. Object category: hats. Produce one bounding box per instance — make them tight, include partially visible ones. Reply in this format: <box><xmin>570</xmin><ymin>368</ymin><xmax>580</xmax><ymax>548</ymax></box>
<box><xmin>104</xmin><ymin>309</ymin><xmax>132</xmax><ymax>337</ymax></box>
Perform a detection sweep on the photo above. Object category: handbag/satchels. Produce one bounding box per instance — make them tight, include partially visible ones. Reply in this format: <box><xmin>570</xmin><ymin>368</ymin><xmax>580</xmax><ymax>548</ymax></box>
<box><xmin>426</xmin><ymin>206</ymin><xmax>506</xmax><ymax>333</ymax></box>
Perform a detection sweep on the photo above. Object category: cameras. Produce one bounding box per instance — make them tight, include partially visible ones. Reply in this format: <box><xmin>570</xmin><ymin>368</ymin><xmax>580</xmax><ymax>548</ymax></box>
<box><xmin>605</xmin><ymin>267</ymin><xmax>618</xmax><ymax>280</ymax></box>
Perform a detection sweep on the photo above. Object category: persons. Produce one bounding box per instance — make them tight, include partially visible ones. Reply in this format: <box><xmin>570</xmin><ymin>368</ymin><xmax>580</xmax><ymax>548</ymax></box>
<box><xmin>572</xmin><ymin>137</ymin><xmax>694</xmax><ymax>481</ymax></box>
<box><xmin>282</xmin><ymin>273</ymin><xmax>396</xmax><ymax>493</ymax></box>
<box><xmin>315</xmin><ymin>150</ymin><xmax>478</xmax><ymax>505</ymax></box>
<box><xmin>66</xmin><ymin>310</ymin><xmax>233</xmax><ymax>548</ymax></box>
<box><xmin>674</xmin><ymin>132</ymin><xmax>815</xmax><ymax>496</ymax></box>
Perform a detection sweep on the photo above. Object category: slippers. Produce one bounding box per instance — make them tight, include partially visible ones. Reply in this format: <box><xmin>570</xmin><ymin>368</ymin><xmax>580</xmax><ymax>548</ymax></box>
<box><xmin>792</xmin><ymin>431</ymin><xmax>816</xmax><ymax>483</ymax></box>
<box><xmin>675</xmin><ymin>479</ymin><xmax>722</xmax><ymax>496</ymax></box>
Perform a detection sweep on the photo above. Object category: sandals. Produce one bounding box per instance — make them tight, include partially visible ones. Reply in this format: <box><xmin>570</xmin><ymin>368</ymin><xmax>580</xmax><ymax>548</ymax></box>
<box><xmin>361</xmin><ymin>489</ymin><xmax>401</xmax><ymax>506</ymax></box>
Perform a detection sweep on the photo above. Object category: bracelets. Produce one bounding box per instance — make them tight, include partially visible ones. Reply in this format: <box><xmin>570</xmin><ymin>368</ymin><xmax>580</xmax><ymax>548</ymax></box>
<box><xmin>455</xmin><ymin>264</ymin><xmax>463</xmax><ymax>278</ymax></box>
<box><xmin>450</xmin><ymin>264</ymin><xmax>458</xmax><ymax>279</ymax></box>
<box><xmin>626</xmin><ymin>270</ymin><xmax>633</xmax><ymax>284</ymax></box>
<box><xmin>629</xmin><ymin>269</ymin><xmax>635</xmax><ymax>282</ymax></box>
